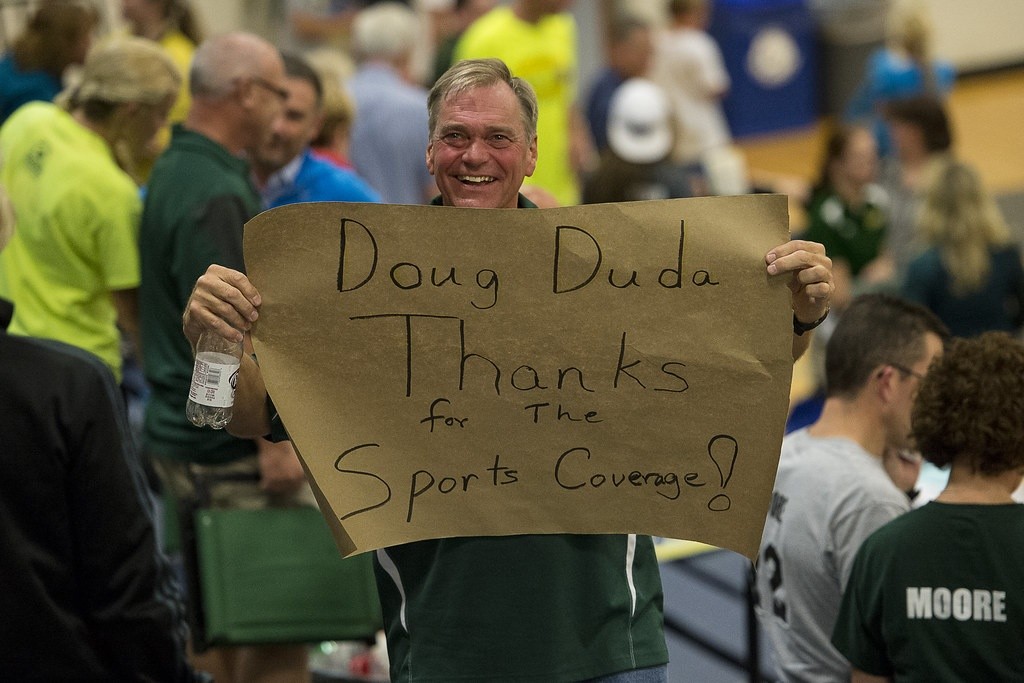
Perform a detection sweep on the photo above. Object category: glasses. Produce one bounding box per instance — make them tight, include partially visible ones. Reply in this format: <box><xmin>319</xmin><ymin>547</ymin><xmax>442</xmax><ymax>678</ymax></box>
<box><xmin>254</xmin><ymin>77</ymin><xmax>288</xmax><ymax>101</ymax></box>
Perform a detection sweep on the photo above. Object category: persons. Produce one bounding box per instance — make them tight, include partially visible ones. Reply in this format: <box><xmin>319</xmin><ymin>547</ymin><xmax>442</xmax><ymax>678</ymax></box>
<box><xmin>584</xmin><ymin>0</ymin><xmax>1024</xmax><ymax>683</ymax></box>
<box><xmin>181</xmin><ymin>63</ymin><xmax>838</xmax><ymax>683</ymax></box>
<box><xmin>0</xmin><ymin>1</ymin><xmax>603</xmax><ymax>683</ymax></box>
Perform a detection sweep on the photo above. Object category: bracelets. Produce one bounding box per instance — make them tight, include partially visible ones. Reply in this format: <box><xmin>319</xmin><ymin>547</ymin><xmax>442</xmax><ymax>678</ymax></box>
<box><xmin>794</xmin><ymin>306</ymin><xmax>831</xmax><ymax>335</ymax></box>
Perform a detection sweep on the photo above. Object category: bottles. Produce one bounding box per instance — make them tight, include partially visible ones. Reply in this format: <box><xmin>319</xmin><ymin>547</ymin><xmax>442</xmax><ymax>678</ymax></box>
<box><xmin>185</xmin><ymin>308</ymin><xmax>245</xmax><ymax>430</ymax></box>
